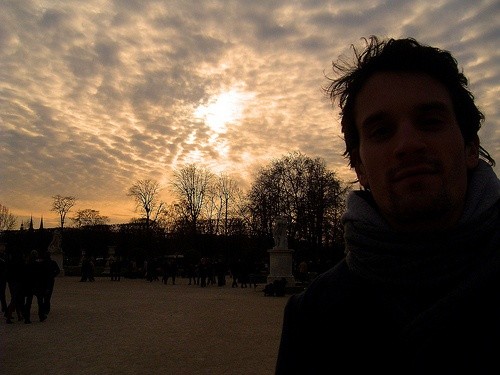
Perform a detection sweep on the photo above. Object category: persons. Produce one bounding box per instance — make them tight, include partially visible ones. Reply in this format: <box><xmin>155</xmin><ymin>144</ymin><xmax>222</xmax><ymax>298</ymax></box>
<box><xmin>273</xmin><ymin>36</ymin><xmax>500</xmax><ymax>375</ymax></box>
<box><xmin>0</xmin><ymin>240</ymin><xmax>321</xmax><ymax>325</ymax></box>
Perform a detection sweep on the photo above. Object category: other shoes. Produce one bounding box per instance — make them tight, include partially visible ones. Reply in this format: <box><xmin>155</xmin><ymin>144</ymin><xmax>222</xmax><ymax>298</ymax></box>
<box><xmin>1</xmin><ymin>308</ymin><xmax>47</xmax><ymax>324</ymax></box>
